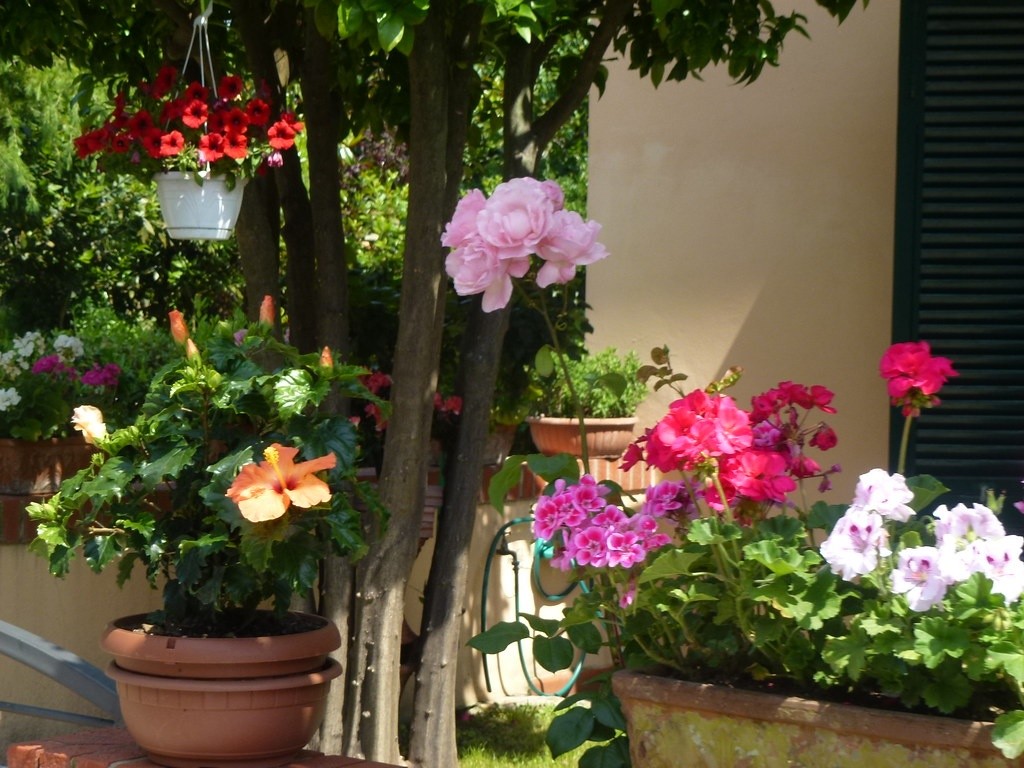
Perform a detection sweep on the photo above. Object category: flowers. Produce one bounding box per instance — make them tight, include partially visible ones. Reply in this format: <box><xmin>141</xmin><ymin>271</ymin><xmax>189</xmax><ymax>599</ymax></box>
<box><xmin>74</xmin><ymin>65</ymin><xmax>304</xmax><ymax>191</ymax></box>
<box><xmin>0</xmin><ymin>330</ymin><xmax>120</xmax><ymax>444</ymax></box>
<box><xmin>24</xmin><ymin>294</ymin><xmax>394</xmax><ymax>634</ymax></box>
<box><xmin>528</xmin><ymin>340</ymin><xmax>1024</xmax><ymax>759</ymax></box>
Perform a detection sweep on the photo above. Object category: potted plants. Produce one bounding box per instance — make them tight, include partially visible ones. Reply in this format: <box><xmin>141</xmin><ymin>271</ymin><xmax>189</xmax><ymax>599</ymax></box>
<box><xmin>524</xmin><ymin>345</ymin><xmax>651</xmax><ymax>458</ymax></box>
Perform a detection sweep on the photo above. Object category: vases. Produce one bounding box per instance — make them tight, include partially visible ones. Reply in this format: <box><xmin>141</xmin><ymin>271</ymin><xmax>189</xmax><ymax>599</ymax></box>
<box><xmin>0</xmin><ymin>435</ymin><xmax>110</xmax><ymax>496</ymax></box>
<box><xmin>612</xmin><ymin>669</ymin><xmax>1024</xmax><ymax>768</ymax></box>
<box><xmin>153</xmin><ymin>171</ymin><xmax>244</xmax><ymax>240</ymax></box>
<box><xmin>100</xmin><ymin>611</ymin><xmax>344</xmax><ymax>768</ymax></box>
